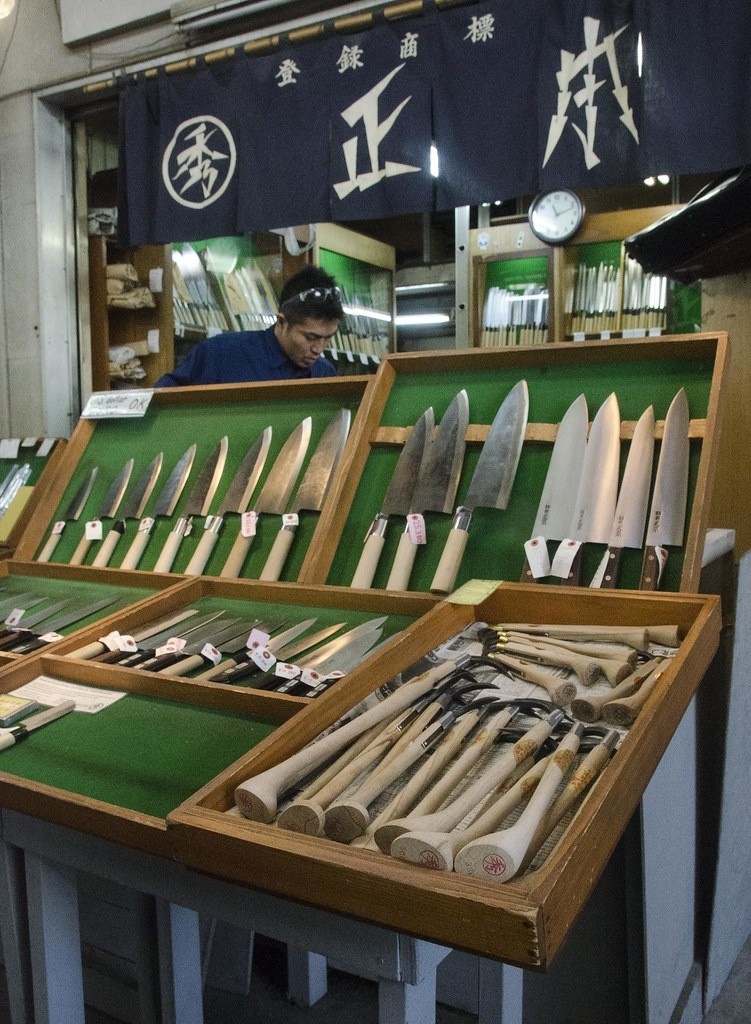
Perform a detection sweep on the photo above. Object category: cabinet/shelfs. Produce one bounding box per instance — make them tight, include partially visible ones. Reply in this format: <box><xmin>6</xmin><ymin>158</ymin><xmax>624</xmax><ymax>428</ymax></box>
<box><xmin>174</xmin><ymin>221</ymin><xmax>397</xmax><ymax>374</ymax></box>
<box><xmin>88</xmin><ymin>226</ymin><xmax>174</xmax><ymax>391</ymax></box>
<box><xmin>468</xmin><ymin>202</ymin><xmax>701</xmax><ymax>347</ymax></box>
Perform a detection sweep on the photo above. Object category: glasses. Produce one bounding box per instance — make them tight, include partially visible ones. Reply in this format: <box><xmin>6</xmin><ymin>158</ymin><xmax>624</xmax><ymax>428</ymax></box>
<box><xmin>282</xmin><ymin>285</ymin><xmax>342</xmax><ymax>310</ymax></box>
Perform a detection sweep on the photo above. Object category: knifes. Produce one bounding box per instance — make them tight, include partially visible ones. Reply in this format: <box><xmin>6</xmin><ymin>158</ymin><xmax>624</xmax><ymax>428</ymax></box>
<box><xmin>639</xmin><ymin>387</ymin><xmax>689</xmax><ymax>591</ymax></box>
<box><xmin>1</xmin><ymin>592</ymin><xmax>121</xmax><ymax>654</ymax></box>
<box><xmin>185</xmin><ymin>426</ymin><xmax>273</xmax><ymax>574</ymax></box>
<box><xmin>385</xmin><ymin>388</ymin><xmax>469</xmax><ymax>591</ymax></box>
<box><xmin>153</xmin><ymin>436</ymin><xmax>229</xmax><ymax>574</ymax></box>
<box><xmin>119</xmin><ymin>445</ymin><xmax>198</xmax><ymax>571</ymax></box>
<box><xmin>258</xmin><ymin>403</ymin><xmax>352</xmax><ymax>583</ymax></box>
<box><xmin>481</xmin><ymin>284</ymin><xmax>549</xmax><ymax>345</ymax></box>
<box><xmin>92</xmin><ymin>451</ymin><xmax>163</xmax><ymax>566</ymax></box>
<box><xmin>223</xmin><ymin>268</ymin><xmax>281</xmax><ymax>331</ymax></box>
<box><xmin>0</xmin><ymin>702</ymin><xmax>76</xmax><ymax>759</ymax></box>
<box><xmin>429</xmin><ymin>378</ymin><xmax>530</xmax><ymax>592</ymax></box>
<box><xmin>69</xmin><ymin>610</ymin><xmax>401</xmax><ymax>699</ymax></box>
<box><xmin>172</xmin><ymin>242</ymin><xmax>231</xmax><ymax>334</ymax></box>
<box><xmin>566</xmin><ymin>253</ymin><xmax>669</xmax><ymax>334</ymax></box>
<box><xmin>69</xmin><ymin>458</ymin><xmax>137</xmax><ymax>564</ymax></box>
<box><xmin>350</xmin><ymin>408</ymin><xmax>435</xmax><ymax>589</ymax></box>
<box><xmin>520</xmin><ymin>392</ymin><xmax>655</xmax><ymax>588</ymax></box>
<box><xmin>220</xmin><ymin>417</ymin><xmax>313</xmax><ymax>578</ymax></box>
<box><xmin>326</xmin><ymin>283</ymin><xmax>389</xmax><ymax>359</ymax></box>
<box><xmin>37</xmin><ymin>466</ymin><xmax>100</xmax><ymax>564</ymax></box>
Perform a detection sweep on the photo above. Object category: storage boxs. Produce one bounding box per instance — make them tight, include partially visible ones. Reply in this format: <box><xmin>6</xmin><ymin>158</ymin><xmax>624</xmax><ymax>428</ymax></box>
<box><xmin>0</xmin><ymin>329</ymin><xmax>733</xmax><ymax>971</ymax></box>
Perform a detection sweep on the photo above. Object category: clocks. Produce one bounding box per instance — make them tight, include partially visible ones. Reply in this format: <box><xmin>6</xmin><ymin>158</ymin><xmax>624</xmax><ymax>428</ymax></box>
<box><xmin>528</xmin><ymin>186</ymin><xmax>586</xmax><ymax>246</ymax></box>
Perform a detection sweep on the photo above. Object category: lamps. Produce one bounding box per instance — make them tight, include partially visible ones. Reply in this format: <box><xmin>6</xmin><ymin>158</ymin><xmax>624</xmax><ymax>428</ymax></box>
<box><xmin>397</xmin><ymin>306</ymin><xmax>456</xmax><ymax>327</ymax></box>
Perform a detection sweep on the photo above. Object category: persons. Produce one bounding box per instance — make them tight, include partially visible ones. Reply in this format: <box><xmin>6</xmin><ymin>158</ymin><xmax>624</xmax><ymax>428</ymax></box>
<box><xmin>154</xmin><ymin>265</ymin><xmax>345</xmax><ymax>388</ymax></box>
